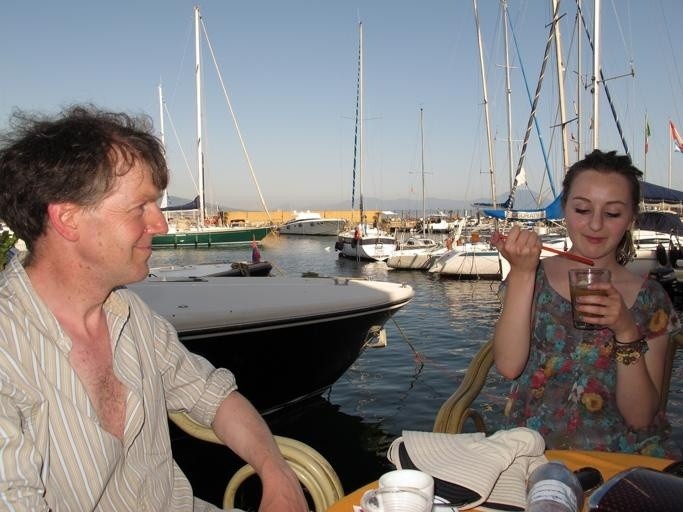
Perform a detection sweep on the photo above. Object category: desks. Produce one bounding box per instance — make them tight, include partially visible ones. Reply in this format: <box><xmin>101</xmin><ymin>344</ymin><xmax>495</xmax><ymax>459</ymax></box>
<box><xmin>306</xmin><ymin>446</ymin><xmax>683</xmax><ymax>512</ymax></box>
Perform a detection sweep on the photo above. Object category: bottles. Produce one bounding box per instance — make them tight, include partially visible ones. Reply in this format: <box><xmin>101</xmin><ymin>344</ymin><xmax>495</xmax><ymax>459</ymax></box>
<box><xmin>525</xmin><ymin>458</ymin><xmax>584</xmax><ymax>512</ymax></box>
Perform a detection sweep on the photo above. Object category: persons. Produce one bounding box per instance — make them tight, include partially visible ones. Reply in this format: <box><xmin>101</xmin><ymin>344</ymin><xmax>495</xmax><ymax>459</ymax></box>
<box><xmin>484</xmin><ymin>149</ymin><xmax>676</xmax><ymax>456</ymax></box>
<box><xmin>0</xmin><ymin>111</ymin><xmax>314</xmax><ymax>512</ymax></box>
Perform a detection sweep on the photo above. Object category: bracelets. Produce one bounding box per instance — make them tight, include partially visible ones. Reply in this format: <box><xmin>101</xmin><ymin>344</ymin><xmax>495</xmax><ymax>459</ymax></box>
<box><xmin>610</xmin><ymin>334</ymin><xmax>649</xmax><ymax>367</ymax></box>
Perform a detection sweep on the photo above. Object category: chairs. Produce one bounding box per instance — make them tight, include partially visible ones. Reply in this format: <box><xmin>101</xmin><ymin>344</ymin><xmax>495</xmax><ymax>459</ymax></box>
<box><xmin>433</xmin><ymin>335</ymin><xmax>500</xmax><ymax>434</ymax></box>
<box><xmin>166</xmin><ymin>402</ymin><xmax>343</xmax><ymax>511</ymax></box>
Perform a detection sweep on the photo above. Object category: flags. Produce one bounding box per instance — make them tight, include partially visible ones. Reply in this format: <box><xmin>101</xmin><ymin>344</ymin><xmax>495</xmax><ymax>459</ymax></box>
<box><xmin>644</xmin><ymin>119</ymin><xmax>651</xmax><ymax>155</ymax></box>
<box><xmin>669</xmin><ymin>120</ymin><xmax>683</xmax><ymax>153</ymax></box>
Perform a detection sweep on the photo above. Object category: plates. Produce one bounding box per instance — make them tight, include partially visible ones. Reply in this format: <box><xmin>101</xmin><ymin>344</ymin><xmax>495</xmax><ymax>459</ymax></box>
<box><xmin>358</xmin><ymin>487</ymin><xmax>458</xmax><ymax>511</ymax></box>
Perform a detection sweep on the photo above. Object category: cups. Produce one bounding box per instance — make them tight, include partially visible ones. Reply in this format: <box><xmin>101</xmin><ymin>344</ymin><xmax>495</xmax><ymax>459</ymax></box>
<box><xmin>568</xmin><ymin>267</ymin><xmax>611</xmax><ymax>330</ymax></box>
<box><xmin>378</xmin><ymin>469</ymin><xmax>435</xmax><ymax>512</ymax></box>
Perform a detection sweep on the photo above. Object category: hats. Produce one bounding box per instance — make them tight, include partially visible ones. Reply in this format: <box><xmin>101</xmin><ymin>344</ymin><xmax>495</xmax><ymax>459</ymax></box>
<box><xmin>386</xmin><ymin>420</ymin><xmax>547</xmax><ymax>512</ymax></box>
